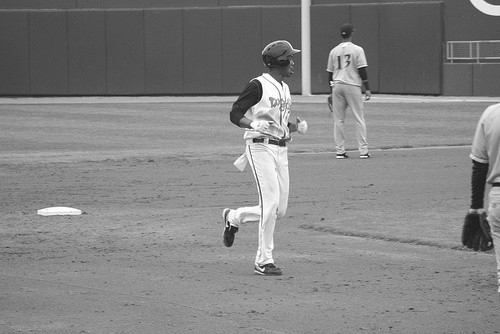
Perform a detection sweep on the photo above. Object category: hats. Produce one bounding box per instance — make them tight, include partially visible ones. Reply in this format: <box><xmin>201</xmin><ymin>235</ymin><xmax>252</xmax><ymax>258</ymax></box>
<box><xmin>340</xmin><ymin>23</ymin><xmax>356</xmax><ymax>33</ymax></box>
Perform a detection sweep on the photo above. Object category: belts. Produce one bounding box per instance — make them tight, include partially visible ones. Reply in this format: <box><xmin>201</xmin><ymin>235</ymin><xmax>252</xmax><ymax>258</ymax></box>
<box><xmin>253</xmin><ymin>138</ymin><xmax>286</xmax><ymax>147</ymax></box>
<box><xmin>492</xmin><ymin>182</ymin><xmax>500</xmax><ymax>187</ymax></box>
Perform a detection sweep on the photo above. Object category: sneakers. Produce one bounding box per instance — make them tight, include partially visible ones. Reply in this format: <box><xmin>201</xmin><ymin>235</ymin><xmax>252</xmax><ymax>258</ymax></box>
<box><xmin>360</xmin><ymin>153</ymin><xmax>370</xmax><ymax>158</ymax></box>
<box><xmin>254</xmin><ymin>262</ymin><xmax>282</xmax><ymax>276</ymax></box>
<box><xmin>336</xmin><ymin>152</ymin><xmax>348</xmax><ymax>158</ymax></box>
<box><xmin>222</xmin><ymin>208</ymin><xmax>238</xmax><ymax>247</ymax></box>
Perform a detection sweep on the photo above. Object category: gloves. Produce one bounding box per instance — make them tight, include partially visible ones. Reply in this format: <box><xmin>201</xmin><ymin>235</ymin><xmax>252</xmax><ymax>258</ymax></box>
<box><xmin>295</xmin><ymin>116</ymin><xmax>308</xmax><ymax>134</ymax></box>
<box><xmin>250</xmin><ymin>120</ymin><xmax>274</xmax><ymax>132</ymax></box>
<box><xmin>365</xmin><ymin>90</ymin><xmax>371</xmax><ymax>100</ymax></box>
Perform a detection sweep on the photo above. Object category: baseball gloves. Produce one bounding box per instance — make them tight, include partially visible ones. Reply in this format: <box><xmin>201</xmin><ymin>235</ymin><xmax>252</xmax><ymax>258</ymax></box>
<box><xmin>461</xmin><ymin>209</ymin><xmax>494</xmax><ymax>252</ymax></box>
<box><xmin>327</xmin><ymin>94</ymin><xmax>333</xmax><ymax>112</ymax></box>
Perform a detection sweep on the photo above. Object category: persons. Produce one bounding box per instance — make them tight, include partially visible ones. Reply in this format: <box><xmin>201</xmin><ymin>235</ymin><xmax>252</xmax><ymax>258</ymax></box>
<box><xmin>325</xmin><ymin>24</ymin><xmax>372</xmax><ymax>158</ymax></box>
<box><xmin>222</xmin><ymin>39</ymin><xmax>308</xmax><ymax>275</ymax></box>
<box><xmin>461</xmin><ymin>104</ymin><xmax>500</xmax><ymax>292</ymax></box>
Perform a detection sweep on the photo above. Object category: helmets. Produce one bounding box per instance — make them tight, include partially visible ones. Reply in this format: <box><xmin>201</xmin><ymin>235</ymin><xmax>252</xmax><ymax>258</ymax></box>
<box><xmin>261</xmin><ymin>40</ymin><xmax>301</xmax><ymax>66</ymax></box>
